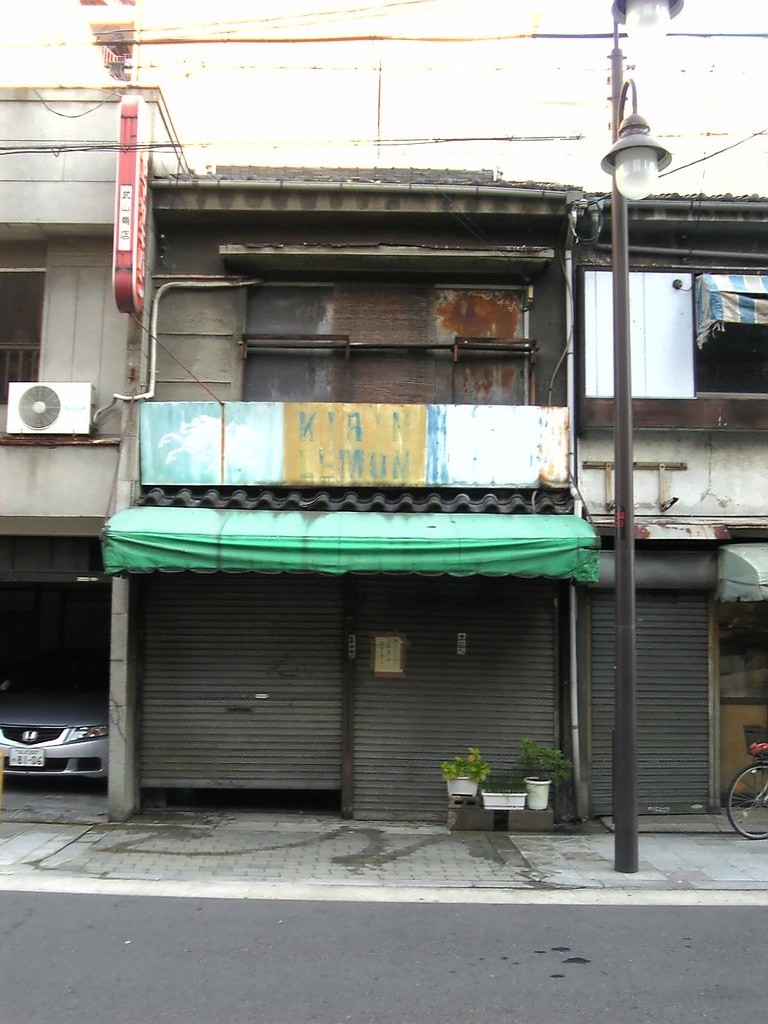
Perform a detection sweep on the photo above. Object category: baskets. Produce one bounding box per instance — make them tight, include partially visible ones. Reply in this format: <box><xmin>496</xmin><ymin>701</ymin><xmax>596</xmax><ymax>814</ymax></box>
<box><xmin>743</xmin><ymin>724</ymin><xmax>768</xmax><ymax>757</ymax></box>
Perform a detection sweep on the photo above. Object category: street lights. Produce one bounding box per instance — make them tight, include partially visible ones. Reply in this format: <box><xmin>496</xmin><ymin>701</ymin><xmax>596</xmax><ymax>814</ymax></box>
<box><xmin>610</xmin><ymin>1</ymin><xmax>685</xmax><ymax>874</ymax></box>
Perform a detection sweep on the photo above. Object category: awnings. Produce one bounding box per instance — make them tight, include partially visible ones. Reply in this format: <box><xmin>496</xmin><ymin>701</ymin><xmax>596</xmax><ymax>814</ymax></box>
<box><xmin>717</xmin><ymin>543</ymin><xmax>768</xmax><ymax>603</ymax></box>
<box><xmin>99</xmin><ymin>505</ymin><xmax>602</xmax><ymax>584</ymax></box>
<box><xmin>693</xmin><ymin>273</ymin><xmax>768</xmax><ymax>349</ymax></box>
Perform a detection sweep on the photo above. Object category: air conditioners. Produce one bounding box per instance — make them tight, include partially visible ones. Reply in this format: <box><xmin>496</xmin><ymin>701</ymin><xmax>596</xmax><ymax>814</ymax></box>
<box><xmin>6</xmin><ymin>381</ymin><xmax>99</xmax><ymax>435</ymax></box>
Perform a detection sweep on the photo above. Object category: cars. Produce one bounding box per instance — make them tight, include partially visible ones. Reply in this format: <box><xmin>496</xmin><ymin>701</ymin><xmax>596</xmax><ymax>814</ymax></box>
<box><xmin>0</xmin><ymin>647</ymin><xmax>110</xmax><ymax>789</ymax></box>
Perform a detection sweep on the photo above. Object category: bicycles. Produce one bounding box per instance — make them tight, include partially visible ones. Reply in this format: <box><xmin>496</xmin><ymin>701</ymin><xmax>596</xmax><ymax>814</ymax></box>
<box><xmin>726</xmin><ymin>724</ymin><xmax>768</xmax><ymax>840</ymax></box>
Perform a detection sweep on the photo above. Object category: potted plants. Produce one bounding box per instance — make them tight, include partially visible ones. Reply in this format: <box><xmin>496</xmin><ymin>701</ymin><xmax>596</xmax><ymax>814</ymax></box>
<box><xmin>517</xmin><ymin>735</ymin><xmax>574</xmax><ymax>811</ymax></box>
<box><xmin>480</xmin><ymin>770</ymin><xmax>537</xmax><ymax>811</ymax></box>
<box><xmin>441</xmin><ymin>747</ymin><xmax>491</xmax><ymax>797</ymax></box>
